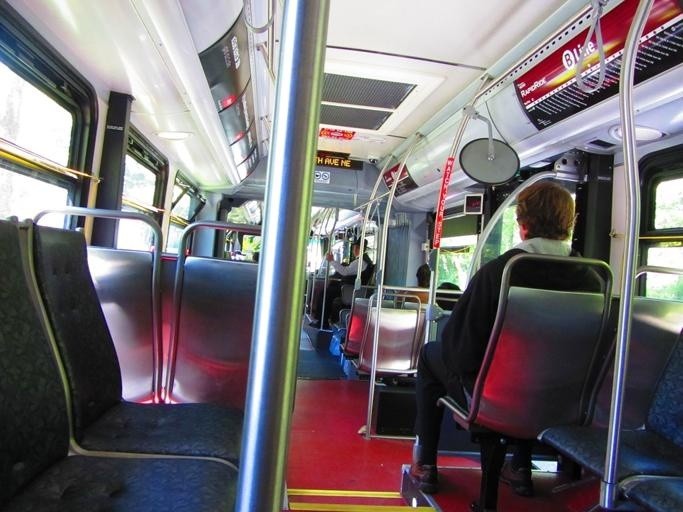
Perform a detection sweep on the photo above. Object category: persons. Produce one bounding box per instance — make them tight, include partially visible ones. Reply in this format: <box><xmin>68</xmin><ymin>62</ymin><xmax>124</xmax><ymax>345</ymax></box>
<box><xmin>396</xmin><ymin>262</ymin><xmax>430</xmax><ymax>304</ymax></box>
<box><xmin>308</xmin><ymin>235</ymin><xmax>374</xmax><ymax>329</ymax></box>
<box><xmin>409</xmin><ymin>178</ymin><xmax>606</xmax><ymax>494</ymax></box>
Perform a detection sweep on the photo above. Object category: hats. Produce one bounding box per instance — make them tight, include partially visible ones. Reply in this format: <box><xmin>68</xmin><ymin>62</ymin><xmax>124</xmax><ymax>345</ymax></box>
<box><xmin>352</xmin><ymin>237</ymin><xmax>371</xmax><ymax>249</ymax></box>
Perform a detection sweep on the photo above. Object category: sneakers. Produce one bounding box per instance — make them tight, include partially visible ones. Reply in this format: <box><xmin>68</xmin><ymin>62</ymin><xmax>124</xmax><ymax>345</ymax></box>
<box><xmin>500</xmin><ymin>461</ymin><xmax>534</xmax><ymax>496</ymax></box>
<box><xmin>409</xmin><ymin>461</ymin><xmax>439</xmax><ymax>494</ymax></box>
<box><xmin>309</xmin><ymin>321</ymin><xmax>329</xmax><ymax>328</ymax></box>
